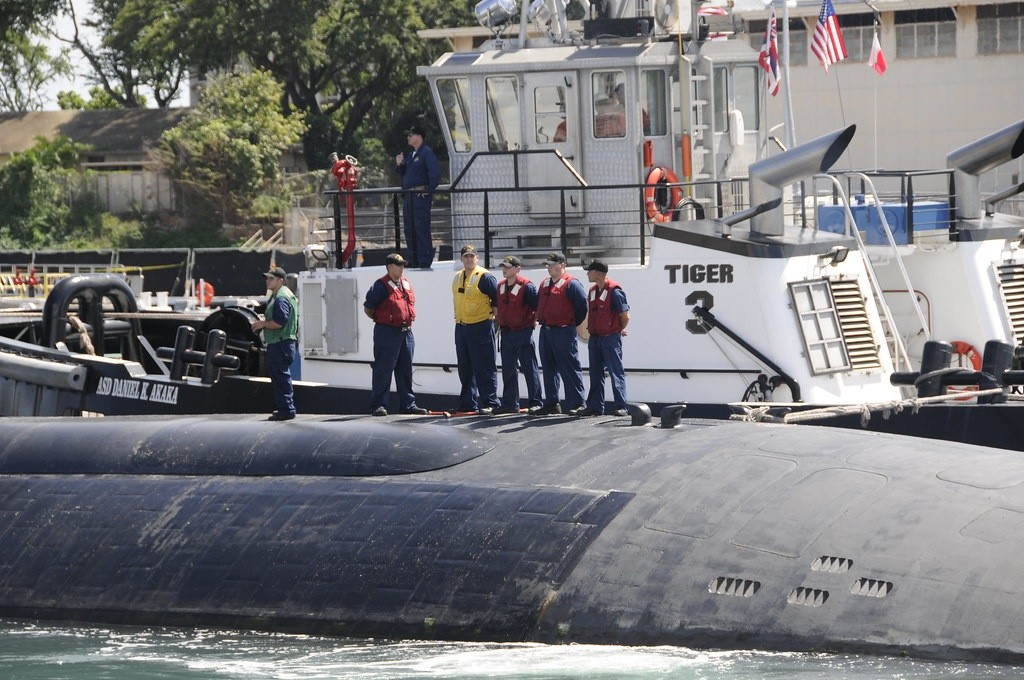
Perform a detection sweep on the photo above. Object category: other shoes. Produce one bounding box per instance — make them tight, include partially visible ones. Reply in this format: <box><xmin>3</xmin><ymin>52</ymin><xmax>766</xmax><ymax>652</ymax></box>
<box><xmin>406</xmin><ymin>262</ymin><xmax>430</xmax><ymax>271</ymax></box>
<box><xmin>479</xmin><ymin>403</ymin><xmax>497</xmax><ymax>414</ymax></box>
<box><xmin>372</xmin><ymin>406</ymin><xmax>387</xmax><ymax>416</ymax></box>
<box><xmin>533</xmin><ymin>403</ymin><xmax>562</xmax><ymax>415</ymax></box>
<box><xmin>576</xmin><ymin>407</ymin><xmax>603</xmax><ymax>416</ymax></box>
<box><xmin>491</xmin><ymin>403</ymin><xmax>520</xmax><ymax>414</ymax></box>
<box><xmin>449</xmin><ymin>400</ymin><xmax>479</xmax><ymax>415</ymax></box>
<box><xmin>399</xmin><ymin>406</ymin><xmax>428</xmax><ymax>414</ymax></box>
<box><xmin>615</xmin><ymin>407</ymin><xmax>629</xmax><ymax>416</ymax></box>
<box><xmin>528</xmin><ymin>405</ymin><xmax>541</xmax><ymax>415</ymax></box>
<box><xmin>569</xmin><ymin>405</ymin><xmax>585</xmax><ymax>416</ymax></box>
<box><xmin>268</xmin><ymin>412</ymin><xmax>295</xmax><ymax>421</ymax></box>
<box><xmin>273</xmin><ymin>410</ymin><xmax>278</xmax><ymax>415</ymax></box>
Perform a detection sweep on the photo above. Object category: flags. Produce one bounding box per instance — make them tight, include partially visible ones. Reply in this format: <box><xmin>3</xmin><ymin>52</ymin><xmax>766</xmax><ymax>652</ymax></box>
<box><xmin>759</xmin><ymin>12</ymin><xmax>781</xmax><ymax>98</ymax></box>
<box><xmin>870</xmin><ymin>30</ymin><xmax>887</xmax><ymax>73</ymax></box>
<box><xmin>699</xmin><ymin>2</ymin><xmax>727</xmax><ymax>16</ymax></box>
<box><xmin>811</xmin><ymin>0</ymin><xmax>848</xmax><ymax>71</ymax></box>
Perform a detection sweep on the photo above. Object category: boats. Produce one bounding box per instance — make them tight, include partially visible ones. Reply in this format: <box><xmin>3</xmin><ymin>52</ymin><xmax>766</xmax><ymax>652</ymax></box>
<box><xmin>1</xmin><ymin>0</ymin><xmax>1024</xmax><ymax>664</ymax></box>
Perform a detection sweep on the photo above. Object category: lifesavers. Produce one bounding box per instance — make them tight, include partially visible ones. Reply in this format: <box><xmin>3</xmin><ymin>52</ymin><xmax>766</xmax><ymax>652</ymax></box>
<box><xmin>646</xmin><ymin>166</ymin><xmax>684</xmax><ymax>225</ymax></box>
<box><xmin>196</xmin><ymin>281</ymin><xmax>214</xmax><ymax>303</ymax></box>
<box><xmin>945</xmin><ymin>340</ymin><xmax>983</xmax><ymax>401</ymax></box>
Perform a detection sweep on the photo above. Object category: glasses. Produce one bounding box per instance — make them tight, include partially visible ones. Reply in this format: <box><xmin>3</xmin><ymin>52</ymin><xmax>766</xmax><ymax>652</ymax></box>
<box><xmin>406</xmin><ymin>133</ymin><xmax>420</xmax><ymax>138</ymax></box>
<box><xmin>545</xmin><ymin>264</ymin><xmax>562</xmax><ymax>268</ymax></box>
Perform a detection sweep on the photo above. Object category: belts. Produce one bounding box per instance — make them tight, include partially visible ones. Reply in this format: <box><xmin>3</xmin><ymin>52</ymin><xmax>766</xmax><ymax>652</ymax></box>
<box><xmin>457</xmin><ymin>319</ymin><xmax>488</xmax><ymax>326</ymax></box>
<box><xmin>543</xmin><ymin>325</ymin><xmax>571</xmax><ymax>330</ymax></box>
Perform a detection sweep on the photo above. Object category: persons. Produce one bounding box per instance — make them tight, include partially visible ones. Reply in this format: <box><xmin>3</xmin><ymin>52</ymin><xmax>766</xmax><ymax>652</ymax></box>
<box><xmin>554</xmin><ymin>100</ymin><xmax>566</xmax><ymax>141</ymax></box>
<box><xmin>251</xmin><ymin>267</ymin><xmax>296</xmax><ymax>420</ymax></box>
<box><xmin>450</xmin><ymin>245</ymin><xmax>501</xmax><ymax>414</ymax></box>
<box><xmin>364</xmin><ymin>253</ymin><xmax>427</xmax><ymax>414</ymax></box>
<box><xmin>593</xmin><ymin>93</ymin><xmax>625</xmax><ymax>135</ymax></box>
<box><xmin>615</xmin><ymin>83</ymin><xmax>649</xmax><ymax>130</ymax></box>
<box><xmin>537</xmin><ymin>253</ymin><xmax>586</xmax><ymax>415</ymax></box>
<box><xmin>397</xmin><ymin>125</ymin><xmax>438</xmax><ymax>268</ymax></box>
<box><xmin>577</xmin><ymin>259</ymin><xmax>629</xmax><ymax>416</ymax></box>
<box><xmin>492</xmin><ymin>256</ymin><xmax>542</xmax><ymax>413</ymax></box>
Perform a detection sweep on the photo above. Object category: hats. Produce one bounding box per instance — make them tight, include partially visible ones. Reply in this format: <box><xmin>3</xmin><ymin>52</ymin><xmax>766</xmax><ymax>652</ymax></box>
<box><xmin>385</xmin><ymin>253</ymin><xmax>408</xmax><ymax>269</ymax></box>
<box><xmin>583</xmin><ymin>259</ymin><xmax>608</xmax><ymax>272</ymax></box>
<box><xmin>542</xmin><ymin>253</ymin><xmax>567</xmax><ymax>267</ymax></box>
<box><xmin>403</xmin><ymin>124</ymin><xmax>426</xmax><ymax>139</ymax></box>
<box><xmin>499</xmin><ymin>255</ymin><xmax>521</xmax><ymax>268</ymax></box>
<box><xmin>461</xmin><ymin>246</ymin><xmax>478</xmax><ymax>257</ymax></box>
<box><xmin>262</xmin><ymin>267</ymin><xmax>287</xmax><ymax>283</ymax></box>
